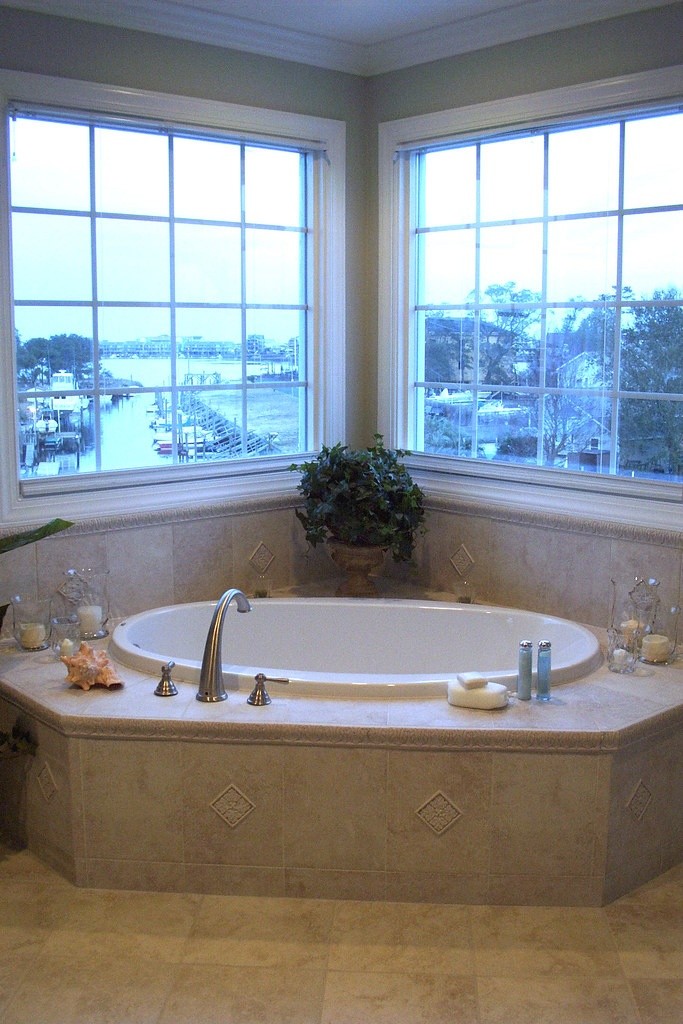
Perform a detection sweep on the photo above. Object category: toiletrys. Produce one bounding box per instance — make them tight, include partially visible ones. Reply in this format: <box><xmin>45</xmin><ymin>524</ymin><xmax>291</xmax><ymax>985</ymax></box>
<box><xmin>517</xmin><ymin>639</ymin><xmax>551</xmax><ymax>701</ymax></box>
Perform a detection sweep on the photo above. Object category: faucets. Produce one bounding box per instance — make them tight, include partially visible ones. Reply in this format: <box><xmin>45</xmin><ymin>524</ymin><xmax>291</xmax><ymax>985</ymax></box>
<box><xmin>196</xmin><ymin>588</ymin><xmax>252</xmax><ymax>703</ymax></box>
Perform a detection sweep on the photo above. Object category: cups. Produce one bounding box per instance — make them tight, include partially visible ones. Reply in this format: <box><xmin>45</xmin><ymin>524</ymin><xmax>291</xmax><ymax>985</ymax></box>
<box><xmin>64</xmin><ymin>566</ymin><xmax>111</xmax><ymax>640</ymax></box>
<box><xmin>10</xmin><ymin>593</ymin><xmax>54</xmax><ymax>651</ymax></box>
<box><xmin>610</xmin><ymin>574</ymin><xmax>661</xmax><ymax>653</ymax></box>
<box><xmin>607</xmin><ymin>628</ymin><xmax>639</xmax><ymax>674</ymax></box>
<box><xmin>638</xmin><ymin>603</ymin><xmax>683</xmax><ymax>664</ymax></box>
<box><xmin>50</xmin><ymin>616</ymin><xmax>81</xmax><ymax>661</ymax></box>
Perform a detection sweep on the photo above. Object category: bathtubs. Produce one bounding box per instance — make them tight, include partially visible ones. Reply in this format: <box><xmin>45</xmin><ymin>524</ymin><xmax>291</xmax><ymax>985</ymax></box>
<box><xmin>100</xmin><ymin>591</ymin><xmax>607</xmax><ymax>702</ymax></box>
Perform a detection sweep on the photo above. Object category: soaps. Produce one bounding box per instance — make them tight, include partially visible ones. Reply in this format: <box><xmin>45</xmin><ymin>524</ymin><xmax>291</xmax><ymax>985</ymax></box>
<box><xmin>457</xmin><ymin>671</ymin><xmax>485</xmax><ymax>690</ymax></box>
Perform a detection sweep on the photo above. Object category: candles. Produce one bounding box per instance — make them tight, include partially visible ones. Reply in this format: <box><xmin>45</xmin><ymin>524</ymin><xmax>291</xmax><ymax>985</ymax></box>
<box><xmin>641</xmin><ymin>634</ymin><xmax>671</xmax><ymax>662</ymax></box>
<box><xmin>614</xmin><ymin>648</ymin><xmax>628</xmax><ymax>665</ymax></box>
<box><xmin>619</xmin><ymin>617</ymin><xmax>645</xmax><ymax>651</ymax></box>
<box><xmin>77</xmin><ymin>601</ymin><xmax>103</xmax><ymax>632</ymax></box>
<box><xmin>60</xmin><ymin>638</ymin><xmax>73</xmax><ymax>657</ymax></box>
<box><xmin>21</xmin><ymin>623</ymin><xmax>46</xmax><ymax>647</ymax></box>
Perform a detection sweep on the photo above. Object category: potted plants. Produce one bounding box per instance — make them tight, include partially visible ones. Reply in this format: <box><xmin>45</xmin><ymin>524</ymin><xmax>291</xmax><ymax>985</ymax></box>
<box><xmin>288</xmin><ymin>434</ymin><xmax>430</xmax><ymax>598</ymax></box>
